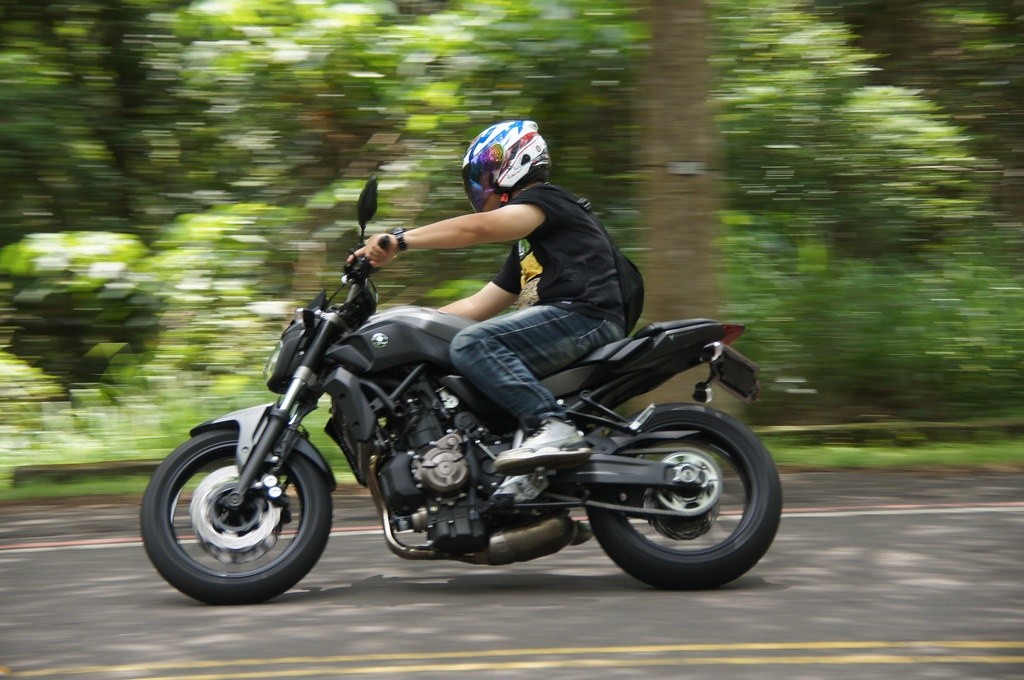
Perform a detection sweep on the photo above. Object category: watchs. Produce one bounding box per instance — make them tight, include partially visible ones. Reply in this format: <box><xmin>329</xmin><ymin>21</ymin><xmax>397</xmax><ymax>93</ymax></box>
<box><xmin>391</xmin><ymin>227</ymin><xmax>408</xmax><ymax>251</ymax></box>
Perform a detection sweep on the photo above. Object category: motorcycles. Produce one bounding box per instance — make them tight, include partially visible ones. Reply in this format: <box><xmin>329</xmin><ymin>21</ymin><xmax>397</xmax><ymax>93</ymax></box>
<box><xmin>138</xmin><ymin>173</ymin><xmax>784</xmax><ymax>608</ymax></box>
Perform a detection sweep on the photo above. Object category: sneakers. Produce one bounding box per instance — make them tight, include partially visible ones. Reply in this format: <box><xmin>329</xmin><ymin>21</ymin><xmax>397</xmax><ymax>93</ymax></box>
<box><xmin>493</xmin><ymin>418</ymin><xmax>593</xmax><ymax>477</ymax></box>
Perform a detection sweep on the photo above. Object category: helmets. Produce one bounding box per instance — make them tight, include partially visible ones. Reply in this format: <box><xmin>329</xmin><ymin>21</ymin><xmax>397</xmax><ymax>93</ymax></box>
<box><xmin>461</xmin><ymin>121</ymin><xmax>551</xmax><ymax>212</ymax></box>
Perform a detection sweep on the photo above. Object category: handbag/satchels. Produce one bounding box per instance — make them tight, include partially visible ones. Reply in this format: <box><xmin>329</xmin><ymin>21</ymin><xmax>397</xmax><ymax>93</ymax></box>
<box><xmin>616</xmin><ymin>254</ymin><xmax>644</xmax><ymax>338</ymax></box>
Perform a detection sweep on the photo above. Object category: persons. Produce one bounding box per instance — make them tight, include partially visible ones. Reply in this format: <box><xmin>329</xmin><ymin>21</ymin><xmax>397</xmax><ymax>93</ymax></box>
<box><xmin>348</xmin><ymin>120</ymin><xmax>627</xmax><ymax>473</ymax></box>
